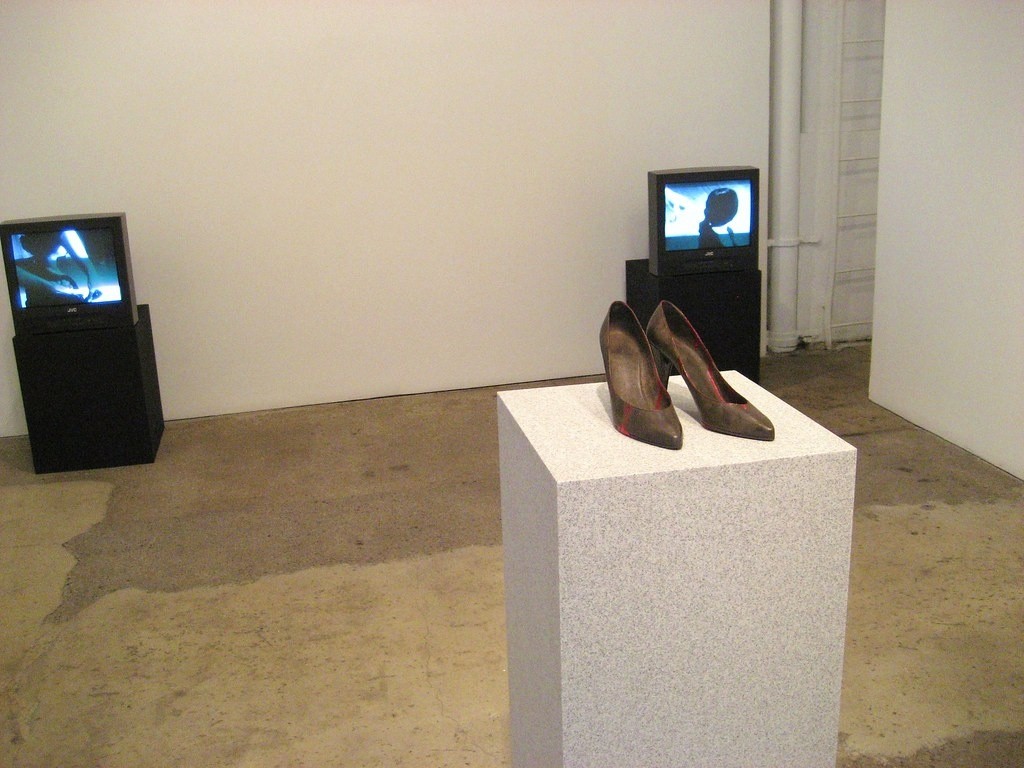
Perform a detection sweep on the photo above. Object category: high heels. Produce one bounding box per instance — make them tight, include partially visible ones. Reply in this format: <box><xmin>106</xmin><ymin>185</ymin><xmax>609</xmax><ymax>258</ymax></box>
<box><xmin>600</xmin><ymin>301</ymin><xmax>775</xmax><ymax>450</ymax></box>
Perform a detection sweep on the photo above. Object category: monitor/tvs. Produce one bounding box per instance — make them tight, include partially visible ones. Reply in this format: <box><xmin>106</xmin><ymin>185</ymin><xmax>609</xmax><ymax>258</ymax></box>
<box><xmin>0</xmin><ymin>212</ymin><xmax>139</xmax><ymax>334</ymax></box>
<box><xmin>649</xmin><ymin>166</ymin><xmax>758</xmax><ymax>277</ymax></box>
